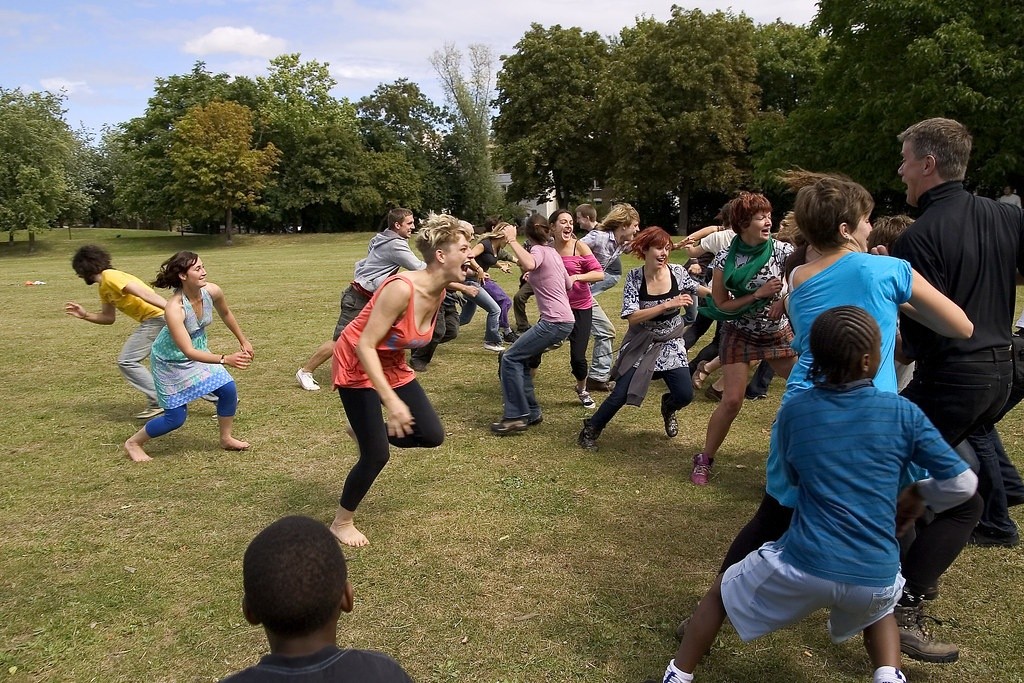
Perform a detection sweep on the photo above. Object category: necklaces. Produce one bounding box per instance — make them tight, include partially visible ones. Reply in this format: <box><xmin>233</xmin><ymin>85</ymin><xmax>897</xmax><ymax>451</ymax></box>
<box><xmin>192</xmin><ymin>298</ymin><xmax>198</xmax><ymax>313</ymax></box>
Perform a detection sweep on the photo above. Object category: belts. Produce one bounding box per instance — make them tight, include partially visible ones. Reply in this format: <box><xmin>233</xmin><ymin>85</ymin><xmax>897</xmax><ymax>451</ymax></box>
<box><xmin>916</xmin><ymin>349</ymin><xmax>1012</xmax><ymax>364</ymax></box>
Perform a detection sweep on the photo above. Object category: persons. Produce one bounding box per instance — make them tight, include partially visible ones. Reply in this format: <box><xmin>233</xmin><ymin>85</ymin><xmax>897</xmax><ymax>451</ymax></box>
<box><xmin>123</xmin><ymin>250</ymin><xmax>256</xmax><ymax>462</ymax></box>
<box><xmin>218</xmin><ymin>515</ymin><xmax>413</xmax><ymax>683</ymax></box>
<box><xmin>295</xmin><ymin>117</ymin><xmax>1024</xmax><ymax>683</ymax></box>
<box><xmin>65</xmin><ymin>245</ymin><xmax>240</xmax><ymax>421</ymax></box>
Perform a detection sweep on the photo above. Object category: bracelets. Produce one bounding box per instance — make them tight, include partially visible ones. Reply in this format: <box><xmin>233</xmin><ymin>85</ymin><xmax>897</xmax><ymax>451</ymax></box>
<box><xmin>752</xmin><ymin>294</ymin><xmax>759</xmax><ymax>300</ymax></box>
<box><xmin>912</xmin><ymin>482</ymin><xmax>933</xmax><ymax>508</ymax></box>
<box><xmin>506</xmin><ymin>237</ymin><xmax>516</xmax><ymax>244</ymax></box>
<box><xmin>476</xmin><ymin>266</ymin><xmax>481</xmax><ymax>271</ymax></box>
<box><xmin>575</xmin><ymin>275</ymin><xmax>578</xmax><ymax>280</ymax></box>
<box><xmin>81</xmin><ymin>311</ymin><xmax>87</xmax><ymax>320</ymax></box>
<box><xmin>499</xmin><ymin>266</ymin><xmax>503</xmax><ymax>270</ymax></box>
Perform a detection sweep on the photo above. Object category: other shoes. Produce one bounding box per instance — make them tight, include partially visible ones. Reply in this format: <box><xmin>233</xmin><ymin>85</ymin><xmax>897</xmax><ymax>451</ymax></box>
<box><xmin>692</xmin><ymin>360</ymin><xmax>711</xmax><ymax>390</ymax></box>
<box><xmin>515</xmin><ymin>325</ymin><xmax>532</xmax><ymax>334</ymax></box>
<box><xmin>409</xmin><ymin>361</ymin><xmax>427</xmax><ymax>372</ymax></box>
<box><xmin>704</xmin><ymin>385</ymin><xmax>724</xmax><ymax>402</ymax></box>
<box><xmin>484</xmin><ymin>341</ymin><xmax>505</xmax><ymax>351</ymax></box>
<box><xmin>967</xmin><ymin>529</ymin><xmax>1020</xmax><ymax>547</ymax></box>
<box><xmin>874</xmin><ymin>666</ymin><xmax>906</xmax><ymax>683</ymax></box>
<box><xmin>920</xmin><ymin>584</ymin><xmax>939</xmax><ymax>601</ymax></box>
<box><xmin>663</xmin><ymin>666</ymin><xmax>684</xmax><ymax>683</ymax></box>
<box><xmin>744</xmin><ymin>393</ymin><xmax>766</xmax><ymax>401</ymax></box>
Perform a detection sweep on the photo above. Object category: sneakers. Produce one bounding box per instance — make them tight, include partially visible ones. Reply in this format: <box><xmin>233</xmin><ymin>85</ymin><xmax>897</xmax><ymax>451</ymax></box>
<box><xmin>135</xmin><ymin>407</ymin><xmax>164</xmax><ymax>419</ymax></box>
<box><xmin>575</xmin><ymin>384</ymin><xmax>596</xmax><ymax>408</ymax></box>
<box><xmin>585</xmin><ymin>376</ymin><xmax>616</xmax><ymax>392</ymax></box>
<box><xmin>691</xmin><ymin>453</ymin><xmax>715</xmax><ymax>484</ymax></box>
<box><xmin>502</xmin><ymin>329</ymin><xmax>520</xmax><ymax>344</ymax></box>
<box><xmin>211</xmin><ymin>397</ymin><xmax>241</xmax><ymax>420</ymax></box>
<box><xmin>295</xmin><ymin>368</ymin><xmax>321</xmax><ymax>391</ymax></box>
<box><xmin>527</xmin><ymin>415</ymin><xmax>543</xmax><ymax>426</ymax></box>
<box><xmin>491</xmin><ymin>417</ymin><xmax>527</xmax><ymax>433</ymax></box>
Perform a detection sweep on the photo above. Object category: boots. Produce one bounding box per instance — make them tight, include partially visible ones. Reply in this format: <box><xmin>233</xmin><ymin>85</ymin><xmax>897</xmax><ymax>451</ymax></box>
<box><xmin>578</xmin><ymin>418</ymin><xmax>599</xmax><ymax>452</ymax></box>
<box><xmin>893</xmin><ymin>600</ymin><xmax>959</xmax><ymax>663</ymax></box>
<box><xmin>661</xmin><ymin>393</ymin><xmax>678</xmax><ymax>438</ymax></box>
<box><xmin>674</xmin><ymin>615</ymin><xmax>692</xmax><ymax>642</ymax></box>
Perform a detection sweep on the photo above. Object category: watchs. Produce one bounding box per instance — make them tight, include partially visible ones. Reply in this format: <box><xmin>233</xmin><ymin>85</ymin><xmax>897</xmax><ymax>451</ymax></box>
<box><xmin>220</xmin><ymin>354</ymin><xmax>225</xmax><ymax>365</ymax></box>
